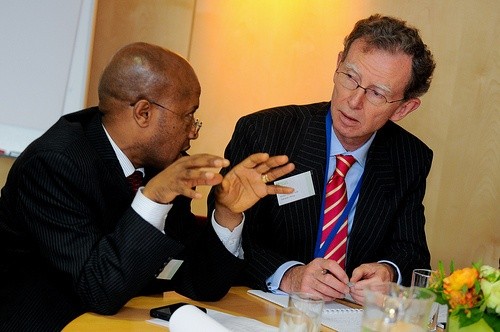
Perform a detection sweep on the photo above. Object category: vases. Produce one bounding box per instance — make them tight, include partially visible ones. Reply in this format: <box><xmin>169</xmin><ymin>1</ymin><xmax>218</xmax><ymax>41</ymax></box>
<box><xmin>446</xmin><ymin>315</ymin><xmax>495</xmax><ymax>332</ymax></box>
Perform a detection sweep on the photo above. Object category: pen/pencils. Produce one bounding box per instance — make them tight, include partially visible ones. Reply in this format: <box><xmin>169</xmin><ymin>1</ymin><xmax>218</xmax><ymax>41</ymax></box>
<box><xmin>321</xmin><ymin>268</ymin><xmax>329</xmax><ymax>275</ymax></box>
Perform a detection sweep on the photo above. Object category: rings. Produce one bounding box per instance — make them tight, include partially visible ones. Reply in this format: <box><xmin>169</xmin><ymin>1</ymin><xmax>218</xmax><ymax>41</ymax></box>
<box><xmin>263</xmin><ymin>173</ymin><xmax>270</xmax><ymax>185</ymax></box>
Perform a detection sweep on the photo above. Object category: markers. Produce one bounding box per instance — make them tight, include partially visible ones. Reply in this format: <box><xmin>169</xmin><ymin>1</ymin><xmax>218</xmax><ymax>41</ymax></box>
<box><xmin>0</xmin><ymin>150</ymin><xmax>20</xmax><ymax>158</ymax></box>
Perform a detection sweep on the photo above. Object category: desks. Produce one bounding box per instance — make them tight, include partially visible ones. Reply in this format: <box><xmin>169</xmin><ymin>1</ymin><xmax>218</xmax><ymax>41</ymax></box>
<box><xmin>60</xmin><ymin>286</ymin><xmax>446</xmax><ymax>332</ymax></box>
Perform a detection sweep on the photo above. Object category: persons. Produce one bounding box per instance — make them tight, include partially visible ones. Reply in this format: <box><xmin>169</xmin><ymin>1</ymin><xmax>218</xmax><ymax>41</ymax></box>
<box><xmin>207</xmin><ymin>14</ymin><xmax>436</xmax><ymax>305</ymax></box>
<box><xmin>0</xmin><ymin>42</ymin><xmax>296</xmax><ymax>332</ymax></box>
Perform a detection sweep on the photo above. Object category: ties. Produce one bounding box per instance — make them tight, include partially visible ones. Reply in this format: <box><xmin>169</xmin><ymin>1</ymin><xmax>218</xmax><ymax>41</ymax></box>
<box><xmin>319</xmin><ymin>155</ymin><xmax>357</xmax><ymax>283</ymax></box>
<box><xmin>127</xmin><ymin>171</ymin><xmax>143</xmax><ymax>200</ymax></box>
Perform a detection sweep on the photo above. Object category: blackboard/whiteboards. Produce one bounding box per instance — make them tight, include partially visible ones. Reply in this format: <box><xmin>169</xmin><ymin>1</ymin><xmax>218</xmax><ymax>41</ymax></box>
<box><xmin>0</xmin><ymin>0</ymin><xmax>98</xmax><ymax>158</ymax></box>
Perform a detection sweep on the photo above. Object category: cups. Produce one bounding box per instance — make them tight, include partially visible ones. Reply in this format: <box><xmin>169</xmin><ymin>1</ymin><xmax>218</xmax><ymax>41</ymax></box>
<box><xmin>278</xmin><ymin>308</ymin><xmax>319</xmax><ymax>332</ymax></box>
<box><xmin>288</xmin><ymin>291</ymin><xmax>325</xmax><ymax>332</ymax></box>
<box><xmin>410</xmin><ymin>269</ymin><xmax>440</xmax><ymax>332</ymax></box>
<box><xmin>361</xmin><ymin>281</ymin><xmax>436</xmax><ymax>332</ymax></box>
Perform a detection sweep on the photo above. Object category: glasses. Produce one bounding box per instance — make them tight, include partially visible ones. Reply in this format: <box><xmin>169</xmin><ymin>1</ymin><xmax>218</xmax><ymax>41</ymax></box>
<box><xmin>334</xmin><ymin>67</ymin><xmax>405</xmax><ymax>106</ymax></box>
<box><xmin>130</xmin><ymin>97</ymin><xmax>202</xmax><ymax>136</ymax></box>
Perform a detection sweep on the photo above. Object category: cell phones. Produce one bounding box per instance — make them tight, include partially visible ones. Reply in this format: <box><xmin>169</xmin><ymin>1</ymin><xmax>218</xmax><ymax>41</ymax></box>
<box><xmin>150</xmin><ymin>302</ymin><xmax>207</xmax><ymax>321</ymax></box>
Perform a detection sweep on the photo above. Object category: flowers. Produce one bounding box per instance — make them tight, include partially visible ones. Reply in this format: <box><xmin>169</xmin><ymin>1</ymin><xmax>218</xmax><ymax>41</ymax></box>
<box><xmin>413</xmin><ymin>257</ymin><xmax>500</xmax><ymax>332</ymax></box>
